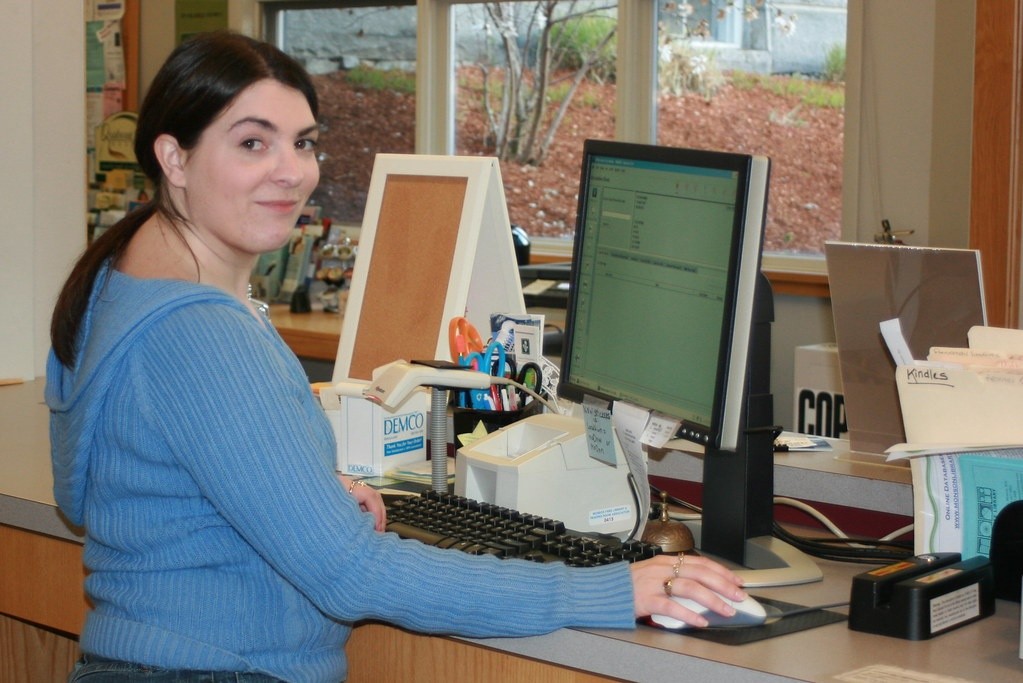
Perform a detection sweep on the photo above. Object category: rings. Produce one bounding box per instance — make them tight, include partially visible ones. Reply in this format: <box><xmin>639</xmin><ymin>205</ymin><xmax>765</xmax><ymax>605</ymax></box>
<box><xmin>664</xmin><ymin>578</ymin><xmax>675</xmax><ymax>597</ymax></box>
<box><xmin>355</xmin><ymin>482</ymin><xmax>366</xmax><ymax>486</ymax></box>
<box><xmin>680</xmin><ymin>553</ymin><xmax>684</xmax><ymax>564</ymax></box>
<box><xmin>349</xmin><ymin>481</ymin><xmax>355</xmax><ymax>495</ymax></box>
<box><xmin>673</xmin><ymin>563</ymin><xmax>679</xmax><ymax>577</ymax></box>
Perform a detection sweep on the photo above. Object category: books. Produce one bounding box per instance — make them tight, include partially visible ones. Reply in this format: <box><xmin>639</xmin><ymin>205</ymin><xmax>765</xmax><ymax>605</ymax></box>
<box><xmin>87</xmin><ymin>168</ymin><xmax>332</xmax><ymax>303</ymax></box>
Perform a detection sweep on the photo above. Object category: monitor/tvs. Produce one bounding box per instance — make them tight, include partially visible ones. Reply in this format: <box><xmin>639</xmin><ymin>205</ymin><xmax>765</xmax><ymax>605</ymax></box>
<box><xmin>557</xmin><ymin>139</ymin><xmax>824</xmax><ymax>588</ymax></box>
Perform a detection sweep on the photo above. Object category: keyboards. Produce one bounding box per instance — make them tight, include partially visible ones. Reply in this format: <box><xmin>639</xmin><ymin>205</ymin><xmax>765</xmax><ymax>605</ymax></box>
<box><xmin>385</xmin><ymin>487</ymin><xmax>664</xmax><ymax>569</ymax></box>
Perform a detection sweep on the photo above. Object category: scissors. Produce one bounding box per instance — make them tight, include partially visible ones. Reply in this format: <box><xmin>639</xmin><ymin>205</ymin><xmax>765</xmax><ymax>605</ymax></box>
<box><xmin>493</xmin><ymin>358</ymin><xmax>543</xmax><ymax>409</ymax></box>
<box><xmin>449</xmin><ymin>317</ymin><xmax>482</xmax><ymax>365</ymax></box>
<box><xmin>466</xmin><ymin>341</ymin><xmax>505</xmax><ymax>412</ymax></box>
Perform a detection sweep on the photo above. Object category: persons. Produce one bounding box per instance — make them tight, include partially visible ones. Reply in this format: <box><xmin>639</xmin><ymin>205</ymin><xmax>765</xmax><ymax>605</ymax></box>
<box><xmin>44</xmin><ymin>32</ymin><xmax>748</xmax><ymax>683</ymax></box>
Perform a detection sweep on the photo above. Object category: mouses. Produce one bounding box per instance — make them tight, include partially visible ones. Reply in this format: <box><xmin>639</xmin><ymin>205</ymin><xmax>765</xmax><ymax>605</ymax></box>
<box><xmin>636</xmin><ymin>586</ymin><xmax>767</xmax><ymax>630</ymax></box>
